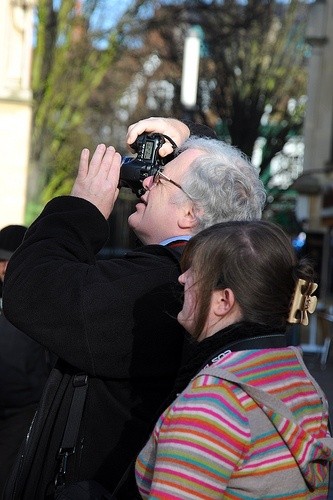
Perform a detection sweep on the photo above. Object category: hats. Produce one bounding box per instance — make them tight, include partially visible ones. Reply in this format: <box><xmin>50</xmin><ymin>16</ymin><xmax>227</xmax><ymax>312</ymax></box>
<box><xmin>0</xmin><ymin>224</ymin><xmax>29</xmax><ymax>260</ymax></box>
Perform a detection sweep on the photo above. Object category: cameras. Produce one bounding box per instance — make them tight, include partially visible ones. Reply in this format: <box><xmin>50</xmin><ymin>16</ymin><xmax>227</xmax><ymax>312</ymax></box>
<box><xmin>118</xmin><ymin>130</ymin><xmax>173</xmax><ymax>198</ymax></box>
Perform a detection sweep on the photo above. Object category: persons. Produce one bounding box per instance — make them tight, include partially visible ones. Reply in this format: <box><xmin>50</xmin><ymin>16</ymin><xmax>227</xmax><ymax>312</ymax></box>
<box><xmin>0</xmin><ymin>224</ymin><xmax>27</xmax><ymax>309</ymax></box>
<box><xmin>0</xmin><ymin>116</ymin><xmax>267</xmax><ymax>500</ymax></box>
<box><xmin>133</xmin><ymin>220</ymin><xmax>332</xmax><ymax>500</ymax></box>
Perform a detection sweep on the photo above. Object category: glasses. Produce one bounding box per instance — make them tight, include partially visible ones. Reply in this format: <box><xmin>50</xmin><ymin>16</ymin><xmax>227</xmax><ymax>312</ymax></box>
<box><xmin>152</xmin><ymin>168</ymin><xmax>196</xmax><ymax>202</ymax></box>
